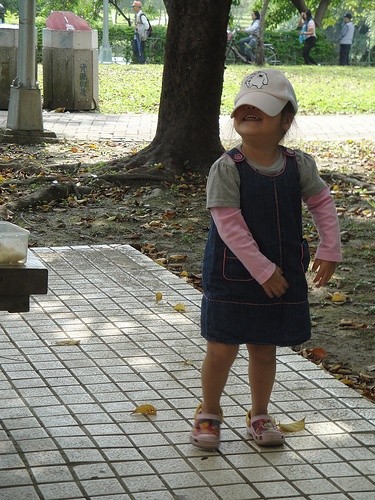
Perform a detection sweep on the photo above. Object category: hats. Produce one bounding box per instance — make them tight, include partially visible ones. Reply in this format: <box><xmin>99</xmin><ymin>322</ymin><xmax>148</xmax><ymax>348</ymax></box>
<box><xmin>130</xmin><ymin>1</ymin><xmax>141</xmax><ymax>6</ymax></box>
<box><xmin>344</xmin><ymin>14</ymin><xmax>352</xmax><ymax>19</ymax></box>
<box><xmin>231</xmin><ymin>68</ymin><xmax>298</xmax><ymax>116</ymax></box>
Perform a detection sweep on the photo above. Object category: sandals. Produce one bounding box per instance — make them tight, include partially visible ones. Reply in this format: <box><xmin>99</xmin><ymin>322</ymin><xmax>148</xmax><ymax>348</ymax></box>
<box><xmin>246</xmin><ymin>409</ymin><xmax>285</xmax><ymax>445</ymax></box>
<box><xmin>190</xmin><ymin>404</ymin><xmax>223</xmax><ymax>450</ymax></box>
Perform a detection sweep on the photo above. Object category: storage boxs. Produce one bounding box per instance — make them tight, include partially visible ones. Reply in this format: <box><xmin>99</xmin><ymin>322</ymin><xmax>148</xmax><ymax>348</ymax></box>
<box><xmin>0</xmin><ymin>221</ymin><xmax>30</xmax><ymax>265</ymax></box>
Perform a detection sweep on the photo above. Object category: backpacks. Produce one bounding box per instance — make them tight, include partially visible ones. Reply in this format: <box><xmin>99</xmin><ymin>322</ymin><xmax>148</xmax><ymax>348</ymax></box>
<box><xmin>140</xmin><ymin>14</ymin><xmax>152</xmax><ymax>37</ymax></box>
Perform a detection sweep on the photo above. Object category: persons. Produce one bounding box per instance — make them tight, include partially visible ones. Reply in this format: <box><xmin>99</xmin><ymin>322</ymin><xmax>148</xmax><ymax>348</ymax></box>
<box><xmin>236</xmin><ymin>10</ymin><xmax>262</xmax><ymax>64</ymax></box>
<box><xmin>298</xmin><ymin>10</ymin><xmax>321</xmax><ymax>67</ymax></box>
<box><xmin>130</xmin><ymin>0</ymin><xmax>153</xmax><ymax>64</ymax></box>
<box><xmin>187</xmin><ymin>69</ymin><xmax>341</xmax><ymax>450</ymax></box>
<box><xmin>338</xmin><ymin>14</ymin><xmax>355</xmax><ymax>66</ymax></box>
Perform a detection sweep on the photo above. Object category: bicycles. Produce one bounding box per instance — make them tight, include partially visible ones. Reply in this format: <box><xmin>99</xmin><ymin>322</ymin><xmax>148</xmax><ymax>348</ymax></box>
<box><xmin>225</xmin><ymin>26</ymin><xmax>280</xmax><ymax>67</ymax></box>
